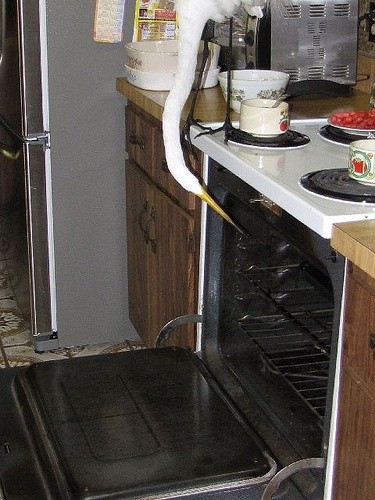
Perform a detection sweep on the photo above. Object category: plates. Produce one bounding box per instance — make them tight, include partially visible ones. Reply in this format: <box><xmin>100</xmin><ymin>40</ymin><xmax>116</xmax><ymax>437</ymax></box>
<box><xmin>122</xmin><ymin>38</ymin><xmax>218</xmax><ymax>91</ymax></box>
<box><xmin>328</xmin><ymin>113</ymin><xmax>375</xmax><ymax>137</ymax></box>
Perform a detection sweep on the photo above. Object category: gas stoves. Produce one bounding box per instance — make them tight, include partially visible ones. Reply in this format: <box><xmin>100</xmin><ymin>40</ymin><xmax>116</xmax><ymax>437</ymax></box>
<box><xmin>224</xmin><ymin>122</ymin><xmax>375</xmax><ymax>207</ymax></box>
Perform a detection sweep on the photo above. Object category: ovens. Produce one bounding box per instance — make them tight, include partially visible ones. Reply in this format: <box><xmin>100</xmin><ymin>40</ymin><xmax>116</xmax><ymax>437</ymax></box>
<box><xmin>7</xmin><ymin>170</ymin><xmax>339</xmax><ymax>500</ymax></box>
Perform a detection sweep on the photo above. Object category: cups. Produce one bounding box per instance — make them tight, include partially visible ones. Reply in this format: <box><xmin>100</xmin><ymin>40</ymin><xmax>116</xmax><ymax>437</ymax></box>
<box><xmin>239</xmin><ymin>100</ymin><xmax>289</xmax><ymax>138</ymax></box>
<box><xmin>346</xmin><ymin>139</ymin><xmax>375</xmax><ymax>188</ymax></box>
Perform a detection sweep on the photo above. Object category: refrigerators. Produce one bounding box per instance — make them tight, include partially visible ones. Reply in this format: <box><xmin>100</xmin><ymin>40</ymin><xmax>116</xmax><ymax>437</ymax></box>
<box><xmin>0</xmin><ymin>1</ymin><xmax>137</xmax><ymax>353</ymax></box>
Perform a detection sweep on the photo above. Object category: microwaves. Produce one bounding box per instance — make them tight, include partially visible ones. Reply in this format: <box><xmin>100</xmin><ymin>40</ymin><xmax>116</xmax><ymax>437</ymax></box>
<box><xmin>201</xmin><ymin>0</ymin><xmax>359</xmax><ymax>94</ymax></box>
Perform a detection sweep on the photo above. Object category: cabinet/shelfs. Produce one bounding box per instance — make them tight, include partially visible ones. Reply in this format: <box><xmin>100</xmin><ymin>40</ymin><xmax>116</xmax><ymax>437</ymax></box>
<box><xmin>328</xmin><ymin>259</ymin><xmax>375</xmax><ymax>500</ymax></box>
<box><xmin>120</xmin><ymin>106</ymin><xmax>202</xmax><ymax>358</ymax></box>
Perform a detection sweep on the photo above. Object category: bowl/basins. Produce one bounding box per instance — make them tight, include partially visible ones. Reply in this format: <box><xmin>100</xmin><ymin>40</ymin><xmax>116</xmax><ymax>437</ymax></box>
<box><xmin>220</xmin><ymin>69</ymin><xmax>290</xmax><ymax>112</ymax></box>
